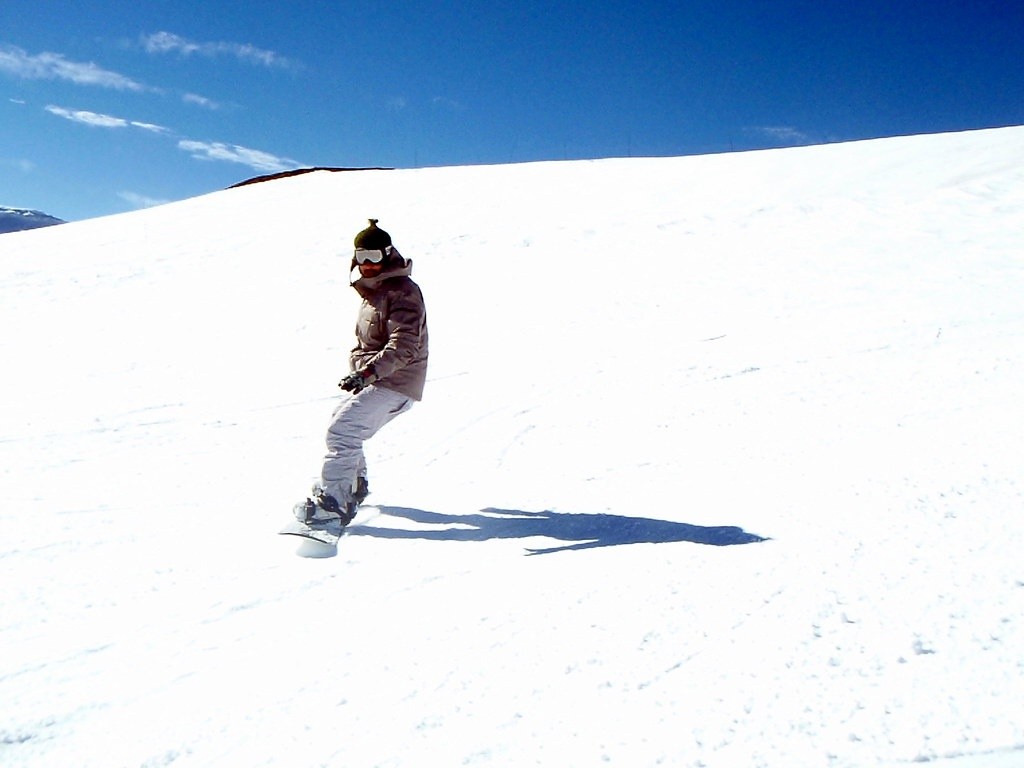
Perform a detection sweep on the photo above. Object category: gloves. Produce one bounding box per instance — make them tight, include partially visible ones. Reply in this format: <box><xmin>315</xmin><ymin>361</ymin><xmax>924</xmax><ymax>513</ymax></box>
<box><xmin>340</xmin><ymin>362</ymin><xmax>379</xmax><ymax>395</ymax></box>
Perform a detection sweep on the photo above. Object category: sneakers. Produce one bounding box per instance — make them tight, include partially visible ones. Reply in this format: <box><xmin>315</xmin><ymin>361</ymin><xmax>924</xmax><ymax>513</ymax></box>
<box><xmin>294</xmin><ymin>477</ymin><xmax>368</xmax><ymax>537</ymax></box>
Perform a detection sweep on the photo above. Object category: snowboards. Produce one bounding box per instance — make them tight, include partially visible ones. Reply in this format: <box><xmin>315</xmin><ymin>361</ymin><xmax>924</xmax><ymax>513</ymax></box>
<box><xmin>279</xmin><ymin>479</ymin><xmax>369</xmax><ymax>544</ymax></box>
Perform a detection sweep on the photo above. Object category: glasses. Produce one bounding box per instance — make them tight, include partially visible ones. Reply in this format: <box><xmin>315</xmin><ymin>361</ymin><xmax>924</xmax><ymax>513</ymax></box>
<box><xmin>355</xmin><ymin>249</ymin><xmax>386</xmax><ymax>266</ymax></box>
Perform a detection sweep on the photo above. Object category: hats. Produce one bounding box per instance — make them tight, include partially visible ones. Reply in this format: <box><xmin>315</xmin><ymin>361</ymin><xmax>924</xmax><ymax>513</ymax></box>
<box><xmin>350</xmin><ymin>218</ymin><xmax>393</xmax><ymax>279</ymax></box>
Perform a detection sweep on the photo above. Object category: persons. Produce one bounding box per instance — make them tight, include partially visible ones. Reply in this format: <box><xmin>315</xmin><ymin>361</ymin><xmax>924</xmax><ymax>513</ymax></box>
<box><xmin>292</xmin><ymin>220</ymin><xmax>428</xmax><ymax>527</ymax></box>
